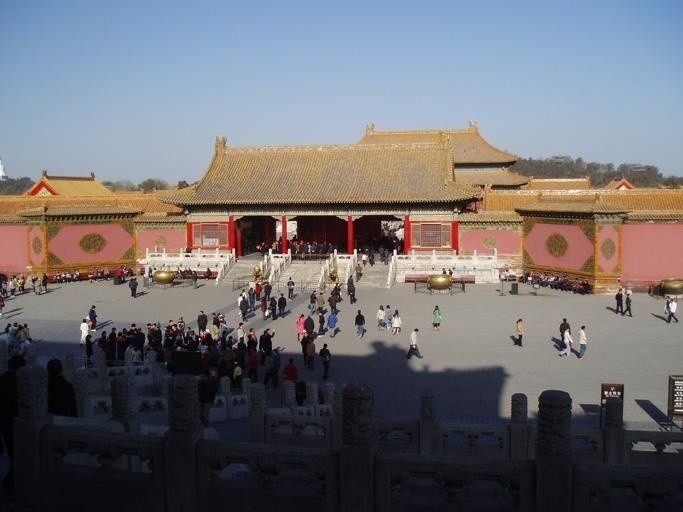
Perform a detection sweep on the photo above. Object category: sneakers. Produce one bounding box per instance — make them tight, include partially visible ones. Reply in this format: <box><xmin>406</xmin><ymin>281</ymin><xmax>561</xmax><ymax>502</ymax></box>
<box><xmin>666</xmin><ymin>319</ymin><xmax>679</xmax><ymax>323</ymax></box>
<box><xmin>303</xmin><ymin>365</ymin><xmax>314</xmax><ymax>370</ymax></box>
<box><xmin>296</xmin><ymin>331</ymin><xmax>335</xmax><ymax>338</ymax></box>
<box><xmin>238</xmin><ymin>297</ymin><xmax>285</xmax><ymax>321</ymax></box>
<box><xmin>378</xmin><ymin>327</ymin><xmax>399</xmax><ymax>335</ymax></box>
<box><xmin>559</xmin><ymin>340</ymin><xmax>586</xmax><ymax>361</ymax></box>
<box><xmin>418</xmin><ymin>356</ymin><xmax>423</xmax><ymax>358</ymax></box>
<box><xmin>357</xmin><ymin>333</ymin><xmax>363</xmax><ymax>337</ymax></box>
<box><xmin>310</xmin><ymin>298</ymin><xmax>355</xmax><ymax>315</ymax></box>
<box><xmin>514</xmin><ymin>342</ymin><xmax>523</xmax><ymax>346</ymax></box>
<box><xmin>323</xmin><ymin>375</ymin><xmax>328</xmax><ymax>380</ymax></box>
<box><xmin>615</xmin><ymin>311</ymin><xmax>633</xmax><ymax>317</ymax></box>
<box><xmin>406</xmin><ymin>356</ymin><xmax>411</xmax><ymax>359</ymax></box>
<box><xmin>433</xmin><ymin>328</ymin><xmax>440</xmax><ymax>334</ymax></box>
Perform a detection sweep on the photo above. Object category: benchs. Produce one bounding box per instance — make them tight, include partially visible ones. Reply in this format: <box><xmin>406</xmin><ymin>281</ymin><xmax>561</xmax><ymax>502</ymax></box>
<box><xmin>404</xmin><ymin>273</ymin><xmax>475</xmax><ymax>284</ymax></box>
<box><xmin>46</xmin><ymin>268</ymin><xmax>133</xmax><ymax>282</ymax></box>
<box><xmin>172</xmin><ymin>270</ymin><xmax>217</xmax><ymax>280</ymax></box>
<box><xmin>521</xmin><ymin>272</ymin><xmax>593</xmax><ymax>295</ymax></box>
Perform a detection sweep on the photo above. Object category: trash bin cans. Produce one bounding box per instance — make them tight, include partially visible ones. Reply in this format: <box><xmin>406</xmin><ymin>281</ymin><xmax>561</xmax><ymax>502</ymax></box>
<box><xmin>511</xmin><ymin>283</ymin><xmax>519</xmax><ymax>296</ymax></box>
<box><xmin>114</xmin><ymin>275</ymin><xmax>123</xmax><ymax>285</ymax></box>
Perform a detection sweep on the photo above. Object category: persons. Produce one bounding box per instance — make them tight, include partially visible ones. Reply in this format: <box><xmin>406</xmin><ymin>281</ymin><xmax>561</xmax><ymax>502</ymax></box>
<box><xmin>92</xmin><ymin>268</ymin><xmax>110</xmax><ymax>283</ymax></box>
<box><xmin>237</xmin><ymin>275</ymin><xmax>442</xmax><ymax>378</ymax></box>
<box><xmin>514</xmin><ymin>319</ymin><xmax>524</xmax><ymax>347</ymax></box>
<box><xmin>663</xmin><ymin>295</ymin><xmax>679</xmax><ymax>323</ymax></box>
<box><xmin>443</xmin><ymin>268</ymin><xmax>452</xmax><ymax>276</ymax></box>
<box><xmin>176</xmin><ymin>266</ymin><xmax>212</xmax><ymax>289</ymax></box>
<box><xmin>79</xmin><ymin>305</ymin><xmax>326</xmax><ymax>429</ymax></box>
<box><xmin>355</xmin><ymin>230</ymin><xmax>403</xmax><ymax>282</ymax></box>
<box><xmin>558</xmin><ymin>318</ymin><xmax>588</xmax><ymax>360</ymax></box>
<box><xmin>1</xmin><ymin>272</ymin><xmax>48</xmax><ymax>306</ymax></box>
<box><xmin>1</xmin><ymin>321</ymin><xmax>78</xmax><ymax>456</ymax></box>
<box><xmin>55</xmin><ymin>269</ymin><xmax>80</xmax><ymax>283</ymax></box>
<box><xmin>122</xmin><ymin>266</ymin><xmax>156</xmax><ymax>298</ymax></box>
<box><xmin>615</xmin><ymin>288</ymin><xmax>633</xmax><ymax>317</ymax></box>
<box><xmin>504</xmin><ymin>269</ymin><xmax>590</xmax><ymax>295</ymax></box>
<box><xmin>257</xmin><ymin>235</ymin><xmax>334</xmax><ymax>259</ymax></box>
